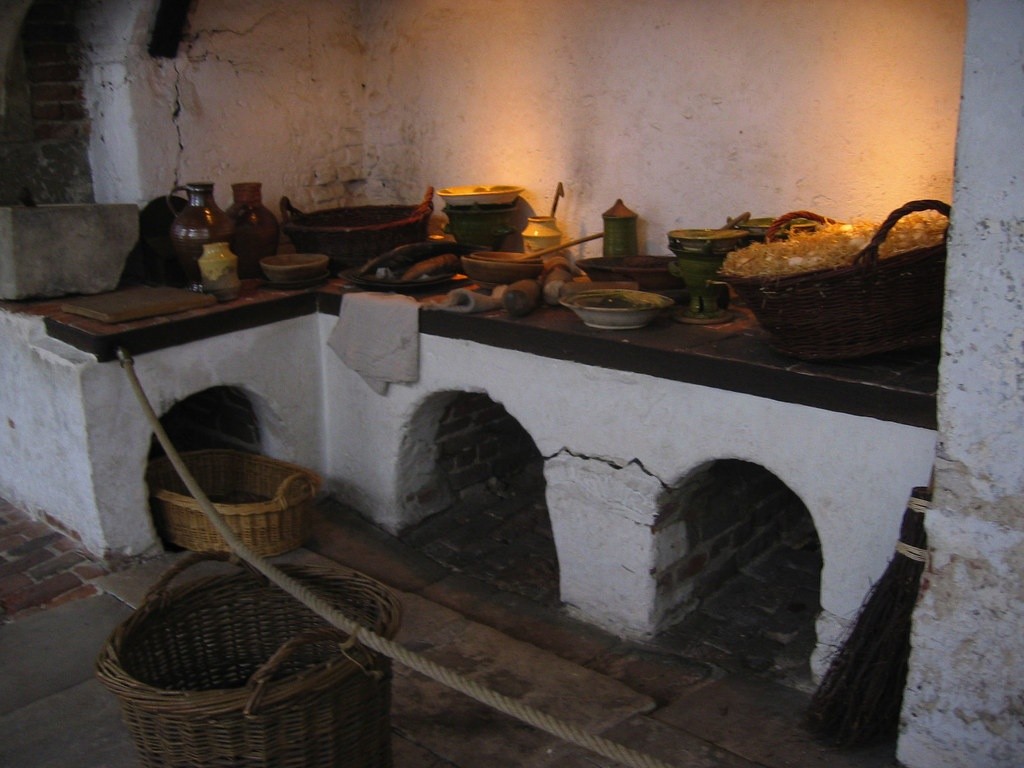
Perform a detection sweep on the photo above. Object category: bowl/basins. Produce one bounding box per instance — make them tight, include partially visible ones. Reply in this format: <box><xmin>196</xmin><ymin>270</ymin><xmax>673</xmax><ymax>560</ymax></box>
<box><xmin>576</xmin><ymin>255</ymin><xmax>683</xmax><ymax>292</ymax></box>
<box><xmin>442</xmin><ymin>207</ymin><xmax>512</xmax><ymax>250</ymax></box>
<box><xmin>259</xmin><ymin>253</ymin><xmax>327</xmax><ymax>291</ymax></box>
<box><xmin>460</xmin><ymin>252</ymin><xmax>545</xmax><ymax>291</ymax></box>
<box><xmin>559</xmin><ymin>289</ymin><xmax>674</xmax><ymax>329</ymax></box>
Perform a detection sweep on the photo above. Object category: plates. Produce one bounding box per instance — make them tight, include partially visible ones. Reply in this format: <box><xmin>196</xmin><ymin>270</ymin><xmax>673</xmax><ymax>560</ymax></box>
<box><xmin>336</xmin><ymin>267</ymin><xmax>456</xmax><ymax>293</ymax></box>
<box><xmin>668</xmin><ymin>228</ymin><xmax>749</xmax><ymax>251</ymax></box>
<box><xmin>436</xmin><ymin>184</ymin><xmax>524</xmax><ymax>206</ymax></box>
<box><xmin>733</xmin><ymin>214</ymin><xmax>820</xmax><ymax>236</ymax></box>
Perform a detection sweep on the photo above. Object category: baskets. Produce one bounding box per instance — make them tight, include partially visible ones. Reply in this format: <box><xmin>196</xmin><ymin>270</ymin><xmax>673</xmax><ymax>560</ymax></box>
<box><xmin>145</xmin><ymin>448</ymin><xmax>320</xmax><ymax>556</ymax></box>
<box><xmin>720</xmin><ymin>198</ymin><xmax>951</xmax><ymax>360</ymax></box>
<box><xmin>98</xmin><ymin>547</ymin><xmax>405</xmax><ymax>767</ymax></box>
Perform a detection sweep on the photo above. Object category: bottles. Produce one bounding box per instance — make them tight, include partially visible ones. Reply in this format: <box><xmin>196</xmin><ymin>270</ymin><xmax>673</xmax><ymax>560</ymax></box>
<box><xmin>168</xmin><ymin>180</ymin><xmax>279</xmax><ymax>301</ymax></box>
<box><xmin>523</xmin><ymin>216</ymin><xmax>564</xmax><ymax>261</ymax></box>
<box><xmin>503</xmin><ymin>279</ymin><xmax>544</xmax><ymax>318</ymax></box>
<box><xmin>601</xmin><ymin>198</ymin><xmax>638</xmax><ymax>259</ymax></box>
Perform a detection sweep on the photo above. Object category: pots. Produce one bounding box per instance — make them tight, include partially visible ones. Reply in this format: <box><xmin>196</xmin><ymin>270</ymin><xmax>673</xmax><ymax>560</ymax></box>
<box><xmin>277</xmin><ymin>187</ymin><xmax>434</xmax><ymax>279</ymax></box>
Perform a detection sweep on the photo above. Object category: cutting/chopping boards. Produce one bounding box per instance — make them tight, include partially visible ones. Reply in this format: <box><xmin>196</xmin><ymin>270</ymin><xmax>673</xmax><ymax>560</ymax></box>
<box><xmin>60</xmin><ymin>287</ymin><xmax>216</xmax><ymax>324</ymax></box>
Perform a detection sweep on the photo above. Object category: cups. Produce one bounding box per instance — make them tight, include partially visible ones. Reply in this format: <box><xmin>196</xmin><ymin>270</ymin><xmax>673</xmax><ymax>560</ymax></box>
<box><xmin>670</xmin><ymin>245</ymin><xmax>733</xmax><ymax>324</ymax></box>
